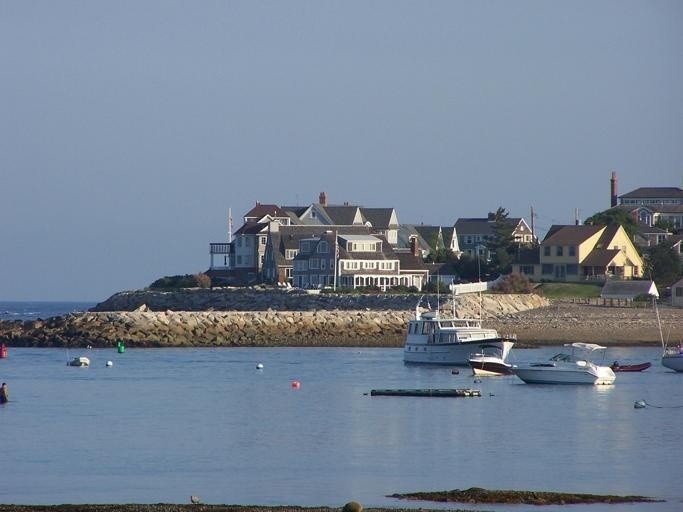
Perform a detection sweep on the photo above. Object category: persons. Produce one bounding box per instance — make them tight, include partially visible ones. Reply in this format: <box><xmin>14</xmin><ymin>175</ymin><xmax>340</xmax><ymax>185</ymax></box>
<box><xmin>0</xmin><ymin>382</ymin><xmax>8</xmax><ymax>404</ymax></box>
